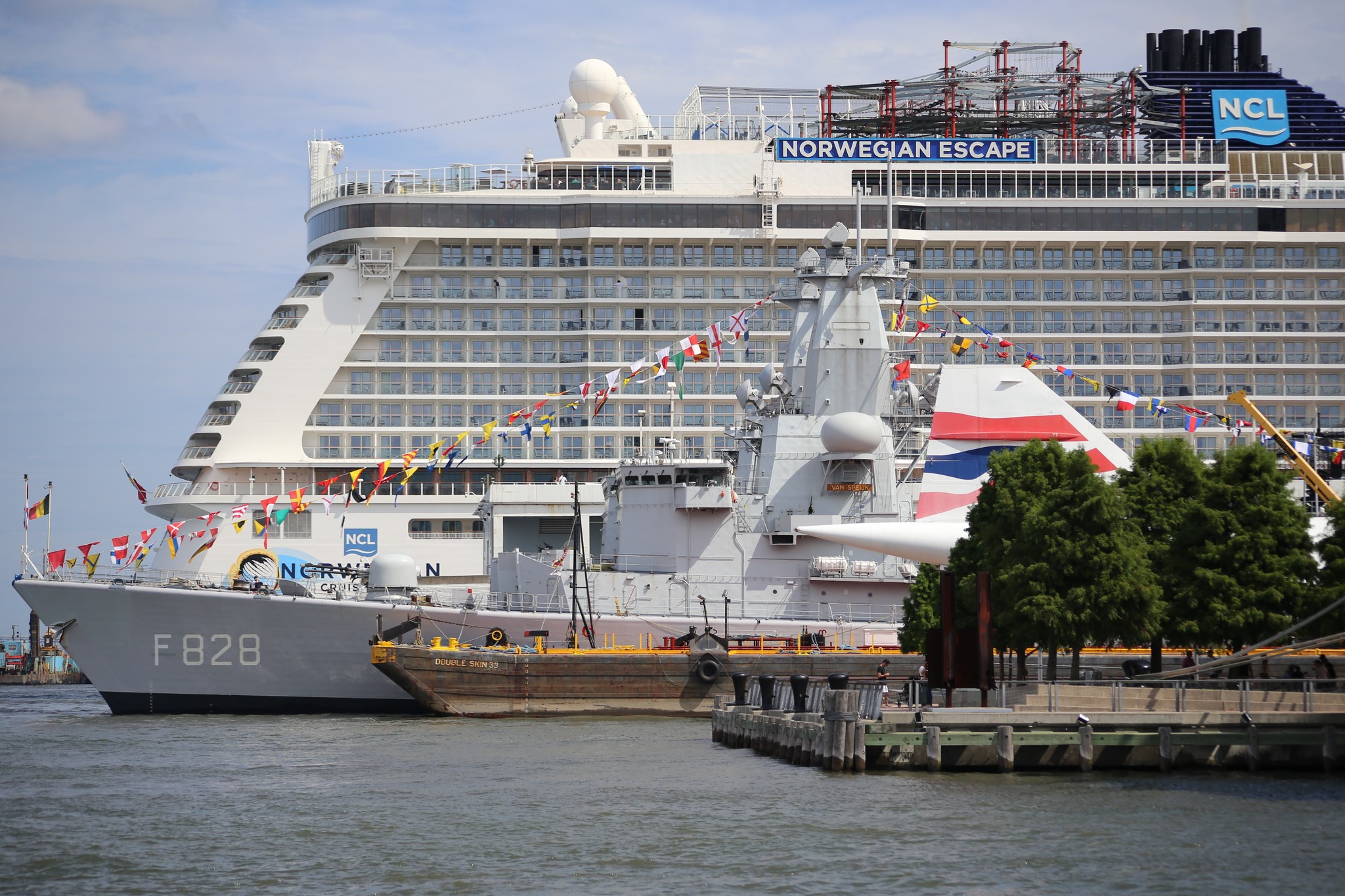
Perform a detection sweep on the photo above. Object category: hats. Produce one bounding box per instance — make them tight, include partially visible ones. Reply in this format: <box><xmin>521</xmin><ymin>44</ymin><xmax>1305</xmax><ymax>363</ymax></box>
<box><xmin>1313</xmin><ymin>660</ymin><xmax>1322</xmax><ymax>665</ymax></box>
<box><xmin>1185</xmin><ymin>651</ymin><xmax>1194</xmax><ymax>655</ymax></box>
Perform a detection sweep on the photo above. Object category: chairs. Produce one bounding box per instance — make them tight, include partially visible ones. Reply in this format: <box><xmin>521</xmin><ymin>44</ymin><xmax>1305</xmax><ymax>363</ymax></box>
<box><xmin>196</xmin><ymin>416</ymin><xmax>231</xmax><ymax>457</ymax></box>
<box><xmin>902</xmin><ymin>188</ymin><xmax>1126</xmax><ymax>198</ymax></box>
<box><xmin>270</xmin><ymin>319</ymin><xmax>296</xmax><ymax>328</ymax></box>
<box><xmin>868</xmin><ymin>259</ymin><xmax>1345</xmax><ymax>430</ymax></box>
<box><xmin>647</xmin><ymin>131</ymin><xmax>655</xmax><ymax>140</ymax></box>
<box><xmin>310</xmin><ymin>255</ymin><xmax>795</xmax><ymax>459</ymax></box>
<box><xmin>714</xmin><ymin>131</ymin><xmax>760</xmax><ymax>140</ymax></box>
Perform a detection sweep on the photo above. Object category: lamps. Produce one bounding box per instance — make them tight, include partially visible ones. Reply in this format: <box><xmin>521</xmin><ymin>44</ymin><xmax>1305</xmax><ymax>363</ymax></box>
<box><xmin>787</xmin><ymin>581</ymin><xmax>795</xmax><ymax>585</ymax></box>
<box><xmin>1079</xmin><ymin>714</ymin><xmax>1089</xmax><ymax>724</ymax></box>
<box><xmin>915</xmin><ymin>712</ymin><xmax>924</xmax><ymax>722</ymax></box>
<box><xmin>688</xmin><ymin>481</ymin><xmax>696</xmax><ymax>487</ymax></box>
<box><xmin>626</xmin><ymin>576</ymin><xmax>635</xmax><ymax>579</ymax></box>
<box><xmin>1242</xmin><ymin>712</ymin><xmax>1253</xmax><ymax>723</ymax></box>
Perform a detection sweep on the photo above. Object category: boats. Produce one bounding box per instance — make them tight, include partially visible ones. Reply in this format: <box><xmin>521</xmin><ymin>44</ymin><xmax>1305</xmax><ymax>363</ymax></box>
<box><xmin>358</xmin><ymin>203</ymin><xmax>1133</xmax><ymax>721</ymax></box>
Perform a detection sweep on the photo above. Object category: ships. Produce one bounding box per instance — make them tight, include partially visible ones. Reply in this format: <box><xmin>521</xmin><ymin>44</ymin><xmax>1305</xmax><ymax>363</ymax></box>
<box><xmin>13</xmin><ymin>26</ymin><xmax>1345</xmax><ymax>716</ymax></box>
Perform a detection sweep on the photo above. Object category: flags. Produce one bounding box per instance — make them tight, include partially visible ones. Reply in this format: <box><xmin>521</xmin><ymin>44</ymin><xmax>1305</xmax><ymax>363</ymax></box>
<box><xmin>894</xmin><ymin>358</ymin><xmax>912</xmax><ymax>381</ymax></box>
<box><xmin>23</xmin><ymin>485</ymin><xmax>29</xmax><ymax>530</ymax></box>
<box><xmin>890</xmin><ymin>279</ymin><xmax>1345</xmax><ymax>471</ymax></box>
<box><xmin>47</xmin><ymin>292</ymin><xmax>774</xmax><ymax>575</ymax></box>
<box><xmin>125</xmin><ymin>472</ymin><xmax>148</xmax><ymax>506</ymax></box>
<box><xmin>552</xmin><ymin>548</ymin><xmax>568</xmax><ymax>571</ymax></box>
<box><xmin>28</xmin><ymin>494</ymin><xmax>49</xmax><ymax>520</ymax></box>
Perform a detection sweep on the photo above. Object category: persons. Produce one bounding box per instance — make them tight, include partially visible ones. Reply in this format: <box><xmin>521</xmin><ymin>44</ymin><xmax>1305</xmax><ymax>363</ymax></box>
<box><xmin>553</xmin><ymin>476</ymin><xmax>559</xmax><ymax>484</ymax></box>
<box><xmin>1240</xmin><ymin>650</ymin><xmax>1338</xmax><ymax>694</ymax></box>
<box><xmin>1182</xmin><ymin>649</ymin><xmax>1195</xmax><ymax>679</ymax></box>
<box><xmin>877</xmin><ymin>659</ymin><xmax>891</xmax><ymax>708</ymax></box>
<box><xmin>251</xmin><ymin>576</ymin><xmax>261</xmax><ymax>589</ymax></box>
<box><xmin>531</xmin><ymin>176</ymin><xmax>641</xmax><ymax>191</ymax></box>
<box><xmin>614</xmin><ymin>280</ymin><xmax>627</xmax><ymax>298</ymax></box>
<box><xmin>1206</xmin><ymin>649</ymin><xmax>1221</xmax><ymax>680</ymax></box>
<box><xmin>920</xmin><ymin>662</ymin><xmax>933</xmax><ymax>706</ymax></box>
<box><xmin>493</xmin><ymin>279</ymin><xmax>500</xmax><ymax>298</ymax></box>
<box><xmin>559</xmin><ymin>474</ymin><xmax>568</xmax><ymax>485</ymax></box>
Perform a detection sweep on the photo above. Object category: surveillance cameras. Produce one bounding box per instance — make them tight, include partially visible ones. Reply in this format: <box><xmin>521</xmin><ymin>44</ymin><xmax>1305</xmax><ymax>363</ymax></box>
<box><xmin>465</xmin><ymin>494</ymin><xmax>467</xmax><ymax>498</ymax></box>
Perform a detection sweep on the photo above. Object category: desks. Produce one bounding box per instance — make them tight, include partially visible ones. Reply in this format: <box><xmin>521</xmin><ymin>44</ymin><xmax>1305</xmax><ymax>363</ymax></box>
<box><xmin>998</xmin><ymin>191</ymin><xmax>1008</xmax><ymax>198</ymax></box>
<box><xmin>912</xmin><ymin>190</ymin><xmax>921</xmax><ymax>197</ymax></box>
<box><xmin>966</xmin><ymin>190</ymin><xmax>976</xmax><ymax>198</ymax></box>
<box><xmin>939</xmin><ymin>191</ymin><xmax>949</xmax><ymax>197</ymax></box>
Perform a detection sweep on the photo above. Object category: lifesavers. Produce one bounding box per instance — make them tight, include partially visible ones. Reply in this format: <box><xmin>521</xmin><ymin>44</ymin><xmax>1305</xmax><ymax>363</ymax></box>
<box><xmin>211</xmin><ymin>482</ymin><xmax>219</xmax><ymax>491</ymax></box>
<box><xmin>510</xmin><ymin>179</ymin><xmax>519</xmax><ymax>188</ymax></box>
<box><xmin>583</xmin><ymin>626</ymin><xmax>595</xmax><ymax>637</ymax></box>
<box><xmin>1230</xmin><ymin>188</ymin><xmax>1239</xmax><ymax>197</ymax></box>
<box><xmin>697</xmin><ymin>661</ymin><xmax>720</xmax><ymax>682</ymax></box>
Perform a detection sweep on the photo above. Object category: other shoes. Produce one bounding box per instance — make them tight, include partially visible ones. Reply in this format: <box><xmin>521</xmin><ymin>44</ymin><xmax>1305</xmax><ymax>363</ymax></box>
<box><xmin>881</xmin><ymin>705</ymin><xmax>891</xmax><ymax>708</ymax></box>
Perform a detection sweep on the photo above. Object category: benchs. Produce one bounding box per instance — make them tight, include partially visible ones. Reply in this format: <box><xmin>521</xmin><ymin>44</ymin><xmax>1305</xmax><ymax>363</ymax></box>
<box><xmin>530</xmin><ymin>182</ymin><xmax>640</xmax><ymax>190</ymax></box>
